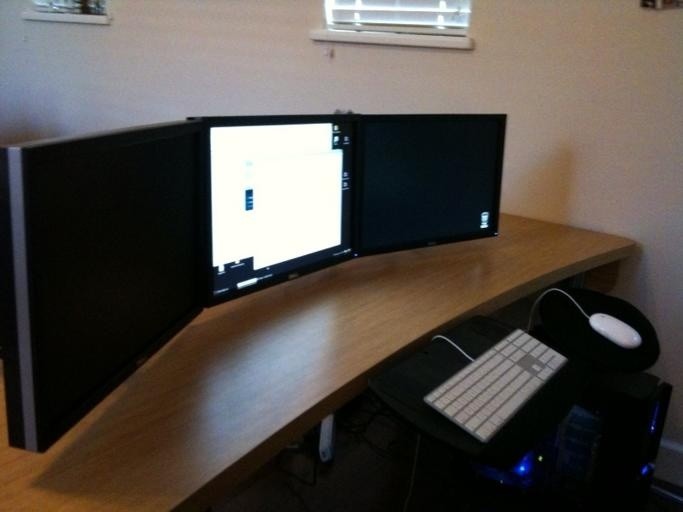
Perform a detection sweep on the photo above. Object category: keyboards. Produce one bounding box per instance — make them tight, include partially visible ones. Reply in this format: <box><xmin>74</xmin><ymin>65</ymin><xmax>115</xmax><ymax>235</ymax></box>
<box><xmin>422</xmin><ymin>327</ymin><xmax>569</xmax><ymax>445</ymax></box>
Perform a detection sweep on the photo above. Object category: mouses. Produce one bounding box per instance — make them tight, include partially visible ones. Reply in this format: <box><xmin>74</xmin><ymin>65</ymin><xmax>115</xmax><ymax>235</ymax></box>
<box><xmin>587</xmin><ymin>313</ymin><xmax>642</xmax><ymax>351</ymax></box>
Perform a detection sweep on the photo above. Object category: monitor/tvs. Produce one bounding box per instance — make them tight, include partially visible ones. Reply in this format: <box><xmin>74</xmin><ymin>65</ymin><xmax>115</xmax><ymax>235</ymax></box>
<box><xmin>351</xmin><ymin>113</ymin><xmax>504</xmax><ymax>258</ymax></box>
<box><xmin>0</xmin><ymin>122</ymin><xmax>207</xmax><ymax>453</ymax></box>
<box><xmin>187</xmin><ymin>113</ymin><xmax>358</xmax><ymax>310</ymax></box>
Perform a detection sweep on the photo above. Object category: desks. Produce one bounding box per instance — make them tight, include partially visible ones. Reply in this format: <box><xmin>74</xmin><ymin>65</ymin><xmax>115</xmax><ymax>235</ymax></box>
<box><xmin>0</xmin><ymin>213</ymin><xmax>636</xmax><ymax>509</ymax></box>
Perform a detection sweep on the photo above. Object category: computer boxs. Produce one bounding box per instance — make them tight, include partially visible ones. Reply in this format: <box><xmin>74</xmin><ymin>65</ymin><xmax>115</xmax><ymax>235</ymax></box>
<box><xmin>567</xmin><ymin>350</ymin><xmax>673</xmax><ymax>510</ymax></box>
